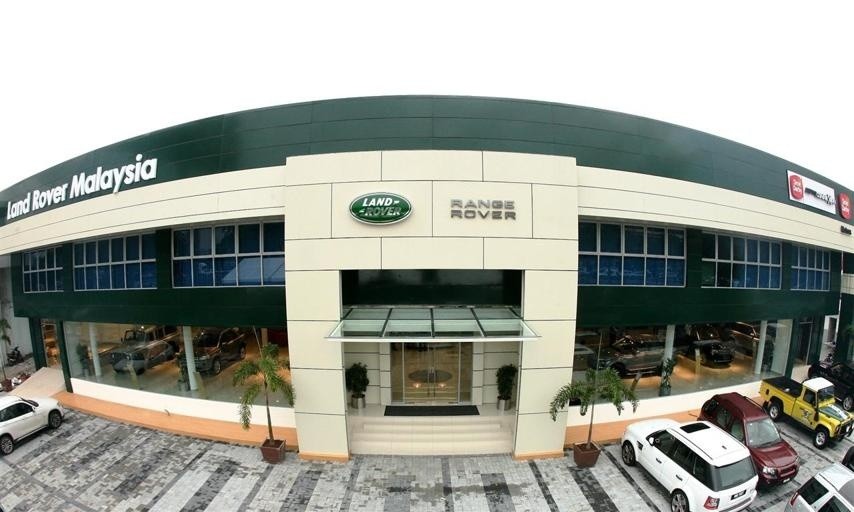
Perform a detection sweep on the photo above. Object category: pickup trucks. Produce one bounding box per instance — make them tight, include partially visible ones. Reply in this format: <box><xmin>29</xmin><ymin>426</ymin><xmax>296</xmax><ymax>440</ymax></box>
<box><xmin>759</xmin><ymin>376</ymin><xmax>854</xmax><ymax>450</ymax></box>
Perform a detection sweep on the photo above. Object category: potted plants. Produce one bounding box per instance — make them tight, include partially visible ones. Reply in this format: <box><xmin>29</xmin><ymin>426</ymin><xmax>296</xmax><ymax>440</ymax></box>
<box><xmin>345</xmin><ymin>362</ymin><xmax>369</xmax><ymax>408</ymax></box>
<box><xmin>172</xmin><ymin>353</ymin><xmax>189</xmax><ymax>391</ymax></box>
<box><xmin>658</xmin><ymin>357</ymin><xmax>678</xmax><ymax>396</ymax></box>
<box><xmin>494</xmin><ymin>364</ymin><xmax>518</xmax><ymax>410</ymax></box>
<box><xmin>230</xmin><ymin>343</ymin><xmax>295</xmax><ymax>464</ymax></box>
<box><xmin>77</xmin><ymin>344</ymin><xmax>91</xmax><ymax>377</ymax></box>
<box><xmin>549</xmin><ymin>368</ymin><xmax>637</xmax><ymax>469</ymax></box>
<box><xmin>0</xmin><ymin>318</ymin><xmax>12</xmax><ymax>392</ymax></box>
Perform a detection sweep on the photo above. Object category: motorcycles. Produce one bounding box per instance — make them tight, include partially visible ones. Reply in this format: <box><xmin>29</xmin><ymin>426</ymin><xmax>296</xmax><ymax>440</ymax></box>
<box><xmin>7</xmin><ymin>346</ymin><xmax>23</xmax><ymax>366</ymax></box>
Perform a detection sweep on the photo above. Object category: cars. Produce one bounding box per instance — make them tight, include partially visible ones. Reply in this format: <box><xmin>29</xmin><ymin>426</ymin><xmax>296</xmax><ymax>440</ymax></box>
<box><xmin>175</xmin><ymin>326</ymin><xmax>246</xmax><ymax>375</ymax></box>
<box><xmin>0</xmin><ymin>394</ymin><xmax>65</xmax><ymax>455</ymax></box>
<box><xmin>572</xmin><ymin>344</ymin><xmax>601</xmax><ymax>396</ymax></box>
<box><xmin>593</xmin><ymin>267</ymin><xmax>677</xmax><ymax>279</ymax></box>
<box><xmin>577</xmin><ymin>322</ymin><xmax>773</xmax><ymax>378</ymax></box>
<box><xmin>750</xmin><ymin>323</ymin><xmax>799</xmax><ymax>352</ymax></box>
<box><xmin>620</xmin><ymin>392</ymin><xmax>854</xmax><ymax>512</ymax></box>
<box><xmin>808</xmin><ymin>361</ymin><xmax>854</xmax><ymax>411</ymax></box>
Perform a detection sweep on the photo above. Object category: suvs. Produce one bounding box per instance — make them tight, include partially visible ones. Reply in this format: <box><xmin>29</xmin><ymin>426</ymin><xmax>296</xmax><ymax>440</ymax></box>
<box><xmin>110</xmin><ymin>326</ymin><xmax>180</xmax><ymax>375</ymax></box>
